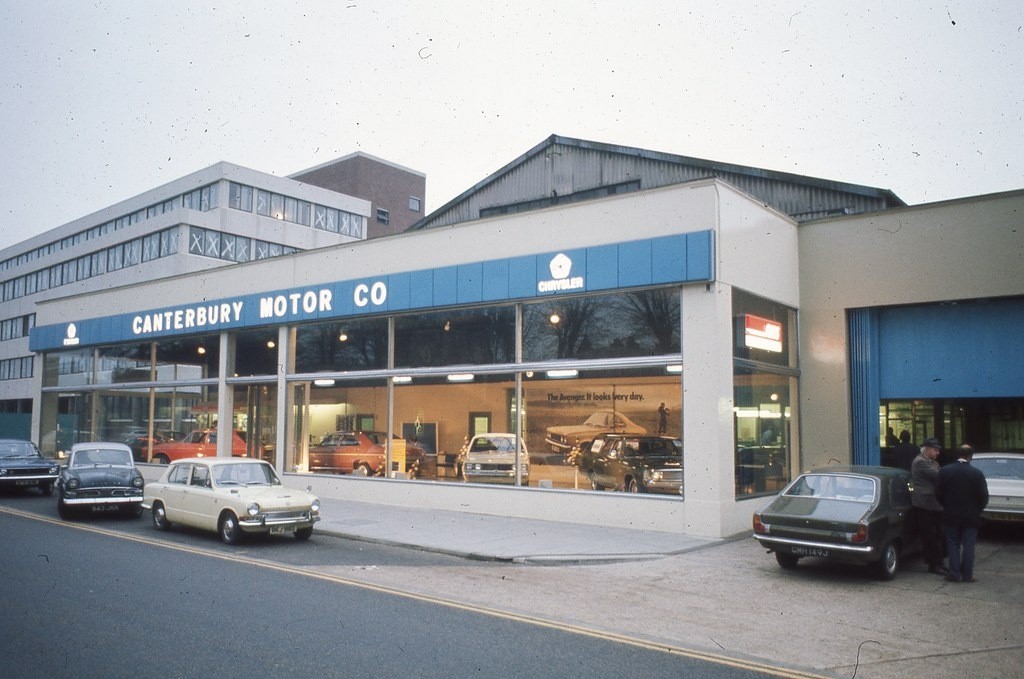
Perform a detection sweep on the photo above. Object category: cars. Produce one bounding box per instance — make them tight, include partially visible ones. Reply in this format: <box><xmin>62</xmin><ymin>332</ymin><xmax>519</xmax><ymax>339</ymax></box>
<box><xmin>0</xmin><ymin>437</ymin><xmax>61</xmax><ymax>495</ymax></box>
<box><xmin>120</xmin><ymin>430</ymin><xmax>183</xmax><ymax>463</ymax></box>
<box><xmin>58</xmin><ymin>442</ymin><xmax>145</xmax><ymax>521</ymax></box>
<box><xmin>578</xmin><ymin>432</ymin><xmax>682</xmax><ymax>494</ymax></box>
<box><xmin>140</xmin><ymin>428</ymin><xmax>268</xmax><ymax>464</ymax></box>
<box><xmin>308</xmin><ymin>428</ymin><xmax>426</xmax><ymax>476</ymax></box>
<box><xmin>970</xmin><ymin>452</ymin><xmax>1024</xmax><ymax>523</ymax></box>
<box><xmin>73</xmin><ymin>431</ymin><xmax>101</xmax><ymax>445</ymax></box>
<box><xmin>141</xmin><ymin>456</ymin><xmax>322</xmax><ymax>546</ymax></box>
<box><xmin>733</xmin><ymin>442</ymin><xmax>790</xmax><ymax>496</ymax></box>
<box><xmin>752</xmin><ymin>465</ymin><xmax>950</xmax><ymax>580</ymax></box>
<box><xmin>453</xmin><ymin>432</ymin><xmax>530</xmax><ymax>487</ymax></box>
<box><xmin>543</xmin><ymin>410</ymin><xmax>648</xmax><ymax>455</ymax></box>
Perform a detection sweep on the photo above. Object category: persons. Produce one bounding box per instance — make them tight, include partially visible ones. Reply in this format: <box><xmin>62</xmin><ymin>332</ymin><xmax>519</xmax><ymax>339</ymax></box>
<box><xmin>663</xmin><ymin>441</ymin><xmax>681</xmax><ymax>455</ymax></box>
<box><xmin>658</xmin><ymin>402</ymin><xmax>670</xmax><ymax>433</ymax></box>
<box><xmin>885</xmin><ymin>427</ymin><xmax>900</xmax><ymax>446</ymax></box>
<box><xmin>935</xmin><ymin>443</ymin><xmax>989</xmax><ymax>583</ymax></box>
<box><xmin>891</xmin><ymin>430</ymin><xmax>921</xmax><ymax>472</ymax></box>
<box><xmin>761</xmin><ymin>426</ymin><xmax>773</xmax><ymax>444</ymax></box>
<box><xmin>911</xmin><ymin>437</ymin><xmax>952</xmax><ymax>576</ymax></box>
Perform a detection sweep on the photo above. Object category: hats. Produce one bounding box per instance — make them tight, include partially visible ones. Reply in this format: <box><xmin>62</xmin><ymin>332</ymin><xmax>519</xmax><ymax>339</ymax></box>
<box><xmin>920</xmin><ymin>437</ymin><xmax>941</xmax><ymax>448</ymax></box>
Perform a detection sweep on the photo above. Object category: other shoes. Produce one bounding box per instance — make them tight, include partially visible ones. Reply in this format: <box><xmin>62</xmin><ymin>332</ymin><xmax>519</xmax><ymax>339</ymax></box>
<box><xmin>929</xmin><ymin>562</ymin><xmax>951</xmax><ymax>576</ymax></box>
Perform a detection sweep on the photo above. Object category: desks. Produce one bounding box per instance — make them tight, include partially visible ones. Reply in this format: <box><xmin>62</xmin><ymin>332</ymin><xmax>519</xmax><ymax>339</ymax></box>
<box><xmin>738</xmin><ymin>464</ymin><xmax>766</xmax><ymax>494</ymax></box>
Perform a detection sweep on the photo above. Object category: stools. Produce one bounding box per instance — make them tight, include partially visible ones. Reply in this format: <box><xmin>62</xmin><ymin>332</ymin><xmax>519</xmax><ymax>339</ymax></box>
<box><xmin>766</xmin><ymin>477</ymin><xmax>790</xmax><ymax>491</ymax></box>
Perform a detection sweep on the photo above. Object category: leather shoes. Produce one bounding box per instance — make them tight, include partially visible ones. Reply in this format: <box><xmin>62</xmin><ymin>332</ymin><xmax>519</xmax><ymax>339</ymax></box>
<box><xmin>944</xmin><ymin>574</ymin><xmax>978</xmax><ymax>583</ymax></box>
